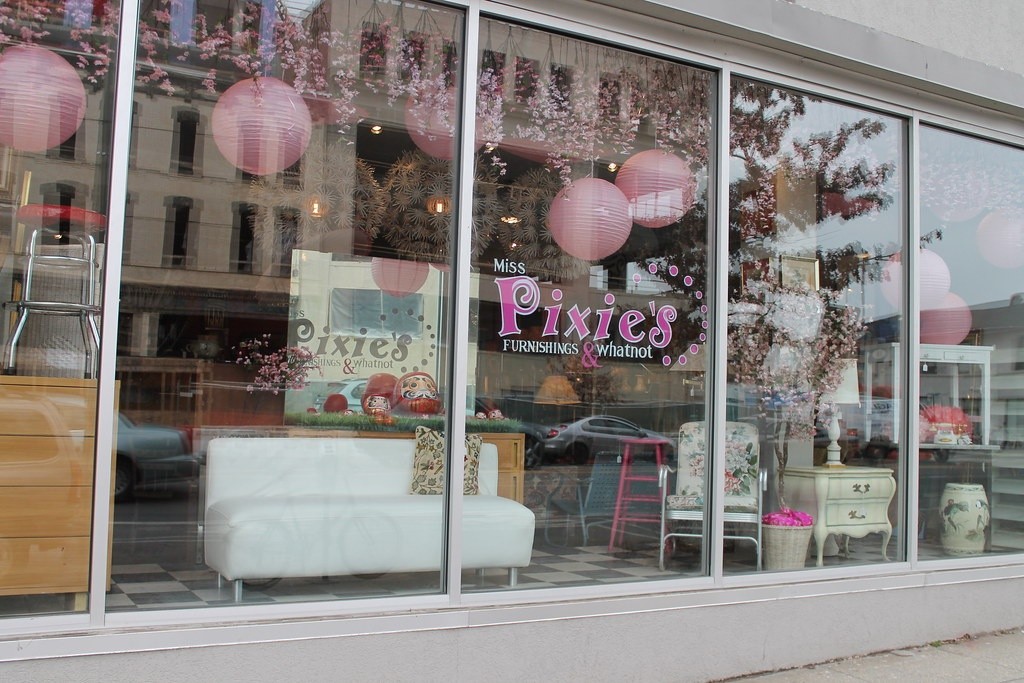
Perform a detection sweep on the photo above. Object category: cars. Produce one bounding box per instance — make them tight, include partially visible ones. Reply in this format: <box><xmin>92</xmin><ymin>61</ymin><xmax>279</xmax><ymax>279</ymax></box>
<box><xmin>736</xmin><ymin>413</ymin><xmax>860</xmax><ymax>467</ymax></box>
<box><xmin>435</xmin><ymin>392</ymin><xmax>545</xmax><ymax>470</ymax></box>
<box><xmin>116</xmin><ymin>412</ymin><xmax>202</xmax><ymax>504</ymax></box>
<box><xmin>538</xmin><ymin>414</ymin><xmax>678</xmax><ymax>467</ymax></box>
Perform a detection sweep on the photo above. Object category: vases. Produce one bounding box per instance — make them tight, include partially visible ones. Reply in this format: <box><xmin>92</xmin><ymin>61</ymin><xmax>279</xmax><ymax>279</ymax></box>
<box><xmin>937</xmin><ymin>482</ymin><xmax>990</xmax><ymax>555</ymax></box>
<box><xmin>760</xmin><ymin>522</ymin><xmax>813</xmax><ymax>570</ymax></box>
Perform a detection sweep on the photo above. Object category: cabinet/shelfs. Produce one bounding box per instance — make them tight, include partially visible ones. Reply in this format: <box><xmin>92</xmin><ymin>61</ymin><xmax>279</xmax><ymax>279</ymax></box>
<box><xmin>774</xmin><ymin>463</ymin><xmax>896</xmax><ymax>567</ymax></box>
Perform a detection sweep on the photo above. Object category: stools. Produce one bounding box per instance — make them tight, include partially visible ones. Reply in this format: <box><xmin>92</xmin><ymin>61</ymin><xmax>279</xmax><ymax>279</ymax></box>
<box><xmin>3</xmin><ymin>203</ymin><xmax>104</xmax><ymax>379</ymax></box>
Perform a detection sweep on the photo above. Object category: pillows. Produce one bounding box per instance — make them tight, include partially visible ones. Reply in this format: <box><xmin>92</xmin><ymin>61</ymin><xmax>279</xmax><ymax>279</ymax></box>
<box><xmin>408</xmin><ymin>424</ymin><xmax>483</xmax><ymax>496</ymax></box>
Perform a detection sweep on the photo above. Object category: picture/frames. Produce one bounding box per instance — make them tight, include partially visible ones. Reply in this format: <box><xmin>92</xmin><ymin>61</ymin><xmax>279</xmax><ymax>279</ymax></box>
<box><xmin>739</xmin><ymin>257</ymin><xmax>773</xmax><ymax>296</ymax></box>
<box><xmin>779</xmin><ymin>254</ymin><xmax>820</xmax><ymax>292</ymax></box>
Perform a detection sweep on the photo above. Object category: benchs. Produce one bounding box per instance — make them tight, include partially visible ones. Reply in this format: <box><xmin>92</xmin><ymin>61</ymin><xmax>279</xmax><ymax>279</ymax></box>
<box><xmin>542</xmin><ymin>449</ymin><xmax>678</xmax><ymax>548</ymax></box>
<box><xmin>202</xmin><ymin>436</ymin><xmax>536</xmax><ymax>605</ymax></box>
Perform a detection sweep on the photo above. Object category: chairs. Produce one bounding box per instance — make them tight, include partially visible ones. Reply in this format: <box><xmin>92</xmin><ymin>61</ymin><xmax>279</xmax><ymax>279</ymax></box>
<box><xmin>605</xmin><ymin>436</ymin><xmax>672</xmax><ymax>561</ymax></box>
<box><xmin>663</xmin><ymin>420</ymin><xmax>767</xmax><ymax>572</ymax></box>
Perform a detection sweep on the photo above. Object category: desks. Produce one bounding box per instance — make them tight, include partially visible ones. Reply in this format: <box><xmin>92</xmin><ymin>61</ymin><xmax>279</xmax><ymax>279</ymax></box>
<box><xmin>853</xmin><ymin>447</ymin><xmax>995</xmax><ymax>545</ymax></box>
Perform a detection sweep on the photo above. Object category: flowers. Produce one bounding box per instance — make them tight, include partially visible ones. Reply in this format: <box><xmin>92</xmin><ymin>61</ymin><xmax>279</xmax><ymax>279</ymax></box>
<box><xmin>723</xmin><ymin>276</ymin><xmax>867</xmax><ymax>510</ymax></box>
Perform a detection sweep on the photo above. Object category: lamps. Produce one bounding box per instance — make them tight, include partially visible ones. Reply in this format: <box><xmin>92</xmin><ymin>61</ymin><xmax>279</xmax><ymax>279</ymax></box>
<box><xmin>810</xmin><ymin>358</ymin><xmax>861</xmax><ymax>467</ymax></box>
<box><xmin>532</xmin><ymin>375</ymin><xmax>583</xmax><ymax>427</ymax></box>
